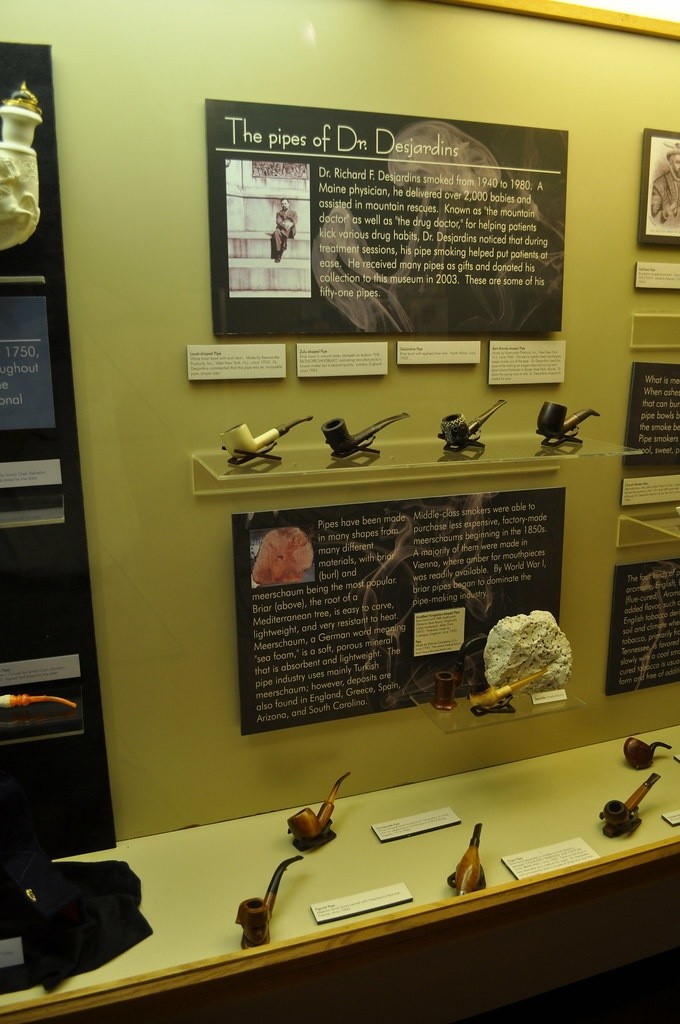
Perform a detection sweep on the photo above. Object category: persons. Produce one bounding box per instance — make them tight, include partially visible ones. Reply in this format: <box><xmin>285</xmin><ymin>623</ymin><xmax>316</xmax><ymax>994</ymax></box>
<box><xmin>271</xmin><ymin>198</ymin><xmax>297</xmax><ymax>263</ymax></box>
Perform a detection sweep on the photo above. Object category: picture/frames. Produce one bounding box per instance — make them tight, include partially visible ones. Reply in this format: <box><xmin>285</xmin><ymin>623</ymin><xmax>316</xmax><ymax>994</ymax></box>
<box><xmin>637</xmin><ymin>128</ymin><xmax>680</xmax><ymax>246</ymax></box>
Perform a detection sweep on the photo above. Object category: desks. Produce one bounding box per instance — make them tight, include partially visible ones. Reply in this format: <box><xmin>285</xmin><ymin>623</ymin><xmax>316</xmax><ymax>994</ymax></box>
<box><xmin>0</xmin><ymin>724</ymin><xmax>680</xmax><ymax>1024</ymax></box>
